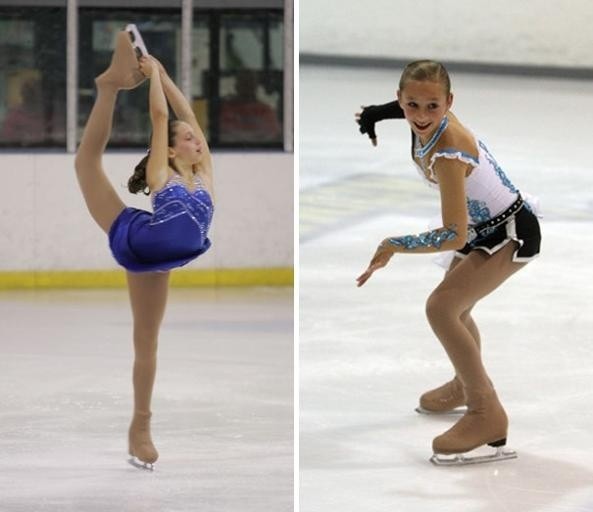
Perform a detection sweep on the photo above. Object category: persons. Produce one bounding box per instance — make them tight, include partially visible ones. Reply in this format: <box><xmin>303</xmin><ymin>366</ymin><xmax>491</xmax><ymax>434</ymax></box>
<box><xmin>73</xmin><ymin>24</ymin><xmax>217</xmax><ymax>470</ymax></box>
<box><xmin>355</xmin><ymin>60</ymin><xmax>541</xmax><ymax>466</ymax></box>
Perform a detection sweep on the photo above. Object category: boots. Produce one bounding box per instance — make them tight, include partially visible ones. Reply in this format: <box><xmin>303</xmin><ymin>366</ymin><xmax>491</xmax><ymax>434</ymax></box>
<box><xmin>420</xmin><ymin>377</ymin><xmax>466</xmax><ymax>412</ymax></box>
<box><xmin>129</xmin><ymin>412</ymin><xmax>157</xmax><ymax>463</ymax></box>
<box><xmin>434</xmin><ymin>390</ymin><xmax>507</xmax><ymax>454</ymax></box>
<box><xmin>94</xmin><ymin>31</ymin><xmax>146</xmax><ymax>91</ymax></box>
